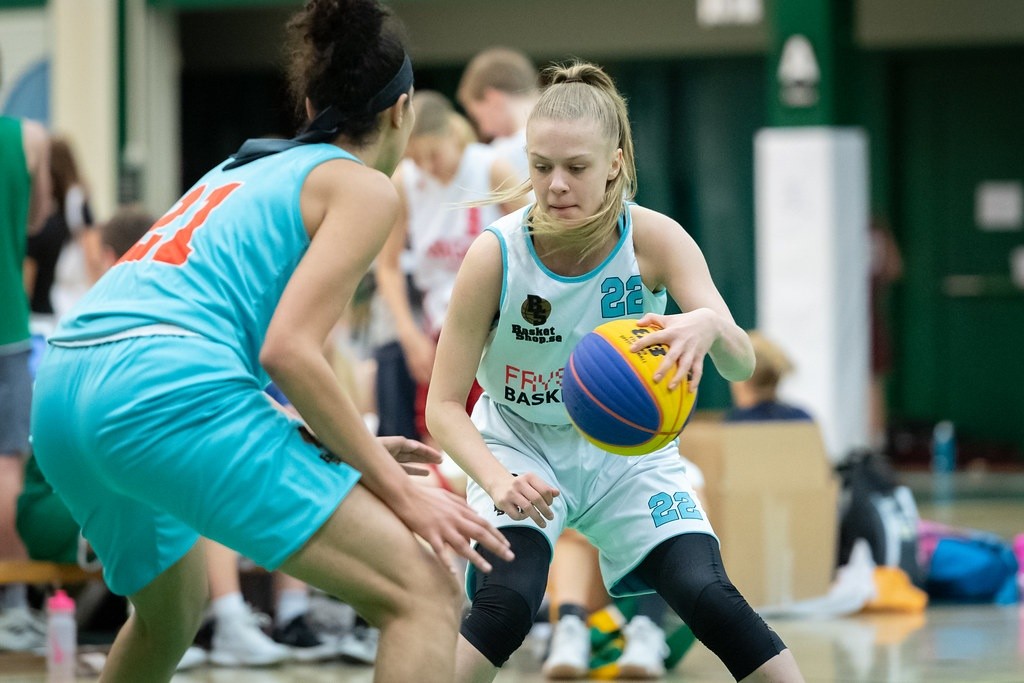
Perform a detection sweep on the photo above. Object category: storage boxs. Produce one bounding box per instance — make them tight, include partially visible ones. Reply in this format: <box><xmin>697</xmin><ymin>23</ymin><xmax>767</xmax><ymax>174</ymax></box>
<box><xmin>668</xmin><ymin>423</ymin><xmax>838</xmax><ymax>612</ymax></box>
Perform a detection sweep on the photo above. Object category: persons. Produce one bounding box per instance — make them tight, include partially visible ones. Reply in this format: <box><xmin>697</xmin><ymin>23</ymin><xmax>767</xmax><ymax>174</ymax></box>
<box><xmin>869</xmin><ymin>220</ymin><xmax>905</xmax><ymax>452</ymax></box>
<box><xmin>1</xmin><ymin>51</ymin><xmax>675</xmax><ymax>683</ymax></box>
<box><xmin>29</xmin><ymin>0</ymin><xmax>515</xmax><ymax>683</ymax></box>
<box><xmin>722</xmin><ymin>330</ymin><xmax>811</xmax><ymax>422</ymax></box>
<box><xmin>425</xmin><ymin>63</ymin><xmax>805</xmax><ymax>683</ymax></box>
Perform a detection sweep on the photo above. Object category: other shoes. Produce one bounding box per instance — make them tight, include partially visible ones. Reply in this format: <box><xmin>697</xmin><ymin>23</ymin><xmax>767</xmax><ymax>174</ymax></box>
<box><xmin>272</xmin><ymin>617</ymin><xmax>334</xmax><ymax>658</ymax></box>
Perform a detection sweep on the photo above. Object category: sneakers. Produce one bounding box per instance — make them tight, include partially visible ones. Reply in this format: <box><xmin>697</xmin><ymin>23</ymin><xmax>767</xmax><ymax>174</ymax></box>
<box><xmin>0</xmin><ymin>608</ymin><xmax>50</xmax><ymax>652</ymax></box>
<box><xmin>209</xmin><ymin>607</ymin><xmax>289</xmax><ymax>665</ymax></box>
<box><xmin>540</xmin><ymin>616</ymin><xmax>591</xmax><ymax>677</ymax></box>
<box><xmin>177</xmin><ymin>647</ymin><xmax>205</xmax><ymax>668</ymax></box>
<box><xmin>618</xmin><ymin>617</ymin><xmax>673</xmax><ymax>679</ymax></box>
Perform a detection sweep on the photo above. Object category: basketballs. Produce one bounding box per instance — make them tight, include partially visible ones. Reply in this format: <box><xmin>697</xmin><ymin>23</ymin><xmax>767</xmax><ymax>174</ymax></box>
<box><xmin>561</xmin><ymin>321</ymin><xmax>702</xmax><ymax>458</ymax></box>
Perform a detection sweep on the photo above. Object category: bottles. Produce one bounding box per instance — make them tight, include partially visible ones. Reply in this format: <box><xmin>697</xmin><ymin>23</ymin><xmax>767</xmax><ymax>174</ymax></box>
<box><xmin>45</xmin><ymin>591</ymin><xmax>78</xmax><ymax>683</ymax></box>
